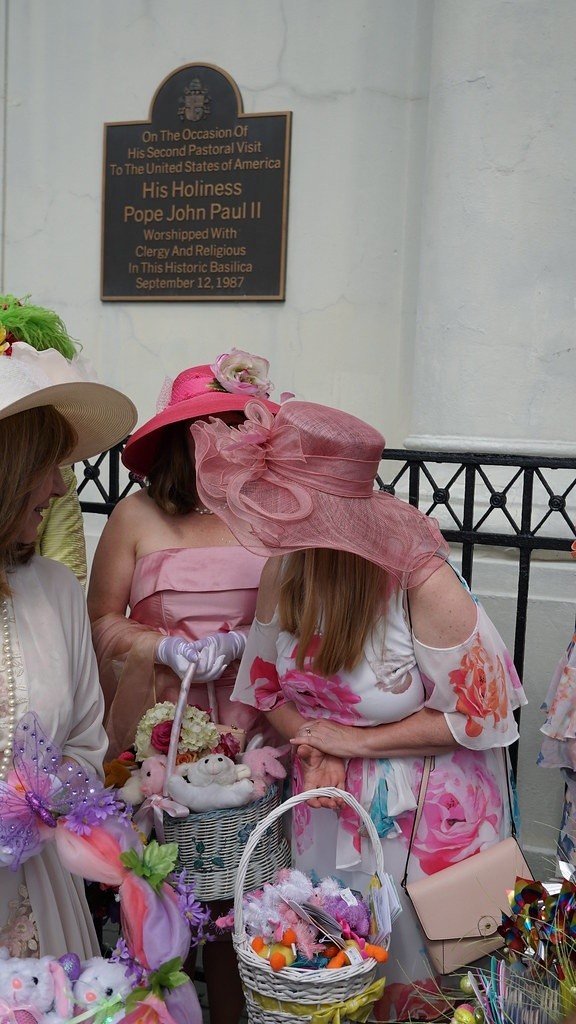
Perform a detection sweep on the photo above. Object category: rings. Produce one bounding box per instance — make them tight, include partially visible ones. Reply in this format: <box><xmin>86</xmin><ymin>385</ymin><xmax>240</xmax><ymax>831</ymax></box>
<box><xmin>306</xmin><ymin>728</ymin><xmax>312</xmax><ymax>738</ymax></box>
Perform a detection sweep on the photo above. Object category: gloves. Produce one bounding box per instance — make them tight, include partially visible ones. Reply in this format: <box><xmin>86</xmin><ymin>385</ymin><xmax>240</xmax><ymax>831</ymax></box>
<box><xmin>154</xmin><ymin>636</ymin><xmax>230</xmax><ymax>685</ymax></box>
<box><xmin>188</xmin><ymin>630</ymin><xmax>248</xmax><ymax>671</ymax></box>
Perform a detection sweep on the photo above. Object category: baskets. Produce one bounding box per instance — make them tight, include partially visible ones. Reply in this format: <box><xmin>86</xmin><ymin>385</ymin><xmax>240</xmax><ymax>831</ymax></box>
<box><xmin>112</xmin><ymin>663</ymin><xmax>293</xmax><ymax>904</ymax></box>
<box><xmin>229</xmin><ymin>791</ymin><xmax>389</xmax><ymax>1024</ymax></box>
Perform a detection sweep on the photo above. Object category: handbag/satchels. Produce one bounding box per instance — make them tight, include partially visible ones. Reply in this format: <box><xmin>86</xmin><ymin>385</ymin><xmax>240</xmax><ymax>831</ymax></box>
<box><xmin>403</xmin><ymin>842</ymin><xmax>532</xmax><ymax>972</ymax></box>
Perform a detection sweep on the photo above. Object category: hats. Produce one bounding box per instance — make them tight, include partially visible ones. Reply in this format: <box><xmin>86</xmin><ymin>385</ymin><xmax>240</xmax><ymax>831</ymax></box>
<box><xmin>0</xmin><ymin>356</ymin><xmax>137</xmax><ymax>472</ymax></box>
<box><xmin>187</xmin><ymin>398</ymin><xmax>453</xmax><ymax>588</ymax></box>
<box><xmin>120</xmin><ymin>350</ymin><xmax>284</xmax><ymax>473</ymax></box>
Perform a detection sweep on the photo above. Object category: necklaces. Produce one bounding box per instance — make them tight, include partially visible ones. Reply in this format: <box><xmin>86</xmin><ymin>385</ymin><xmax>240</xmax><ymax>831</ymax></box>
<box><xmin>190</xmin><ymin>502</ymin><xmax>231</xmax><ymax>516</ymax></box>
<box><xmin>0</xmin><ymin>562</ymin><xmax>19</xmax><ymax>781</ymax></box>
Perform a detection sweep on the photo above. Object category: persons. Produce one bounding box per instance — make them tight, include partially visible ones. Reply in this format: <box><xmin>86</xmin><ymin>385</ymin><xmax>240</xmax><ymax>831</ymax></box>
<box><xmin>0</xmin><ymin>341</ymin><xmax>142</xmax><ymax>1023</ymax></box>
<box><xmin>1</xmin><ymin>292</ymin><xmax>90</xmax><ymax>593</ymax></box>
<box><xmin>537</xmin><ymin>537</ymin><xmax>575</xmax><ymax>883</ymax></box>
<box><xmin>87</xmin><ymin>345</ymin><xmax>285</xmax><ymax>1019</ymax></box>
<box><xmin>184</xmin><ymin>390</ymin><xmax>527</xmax><ymax>1021</ymax></box>
